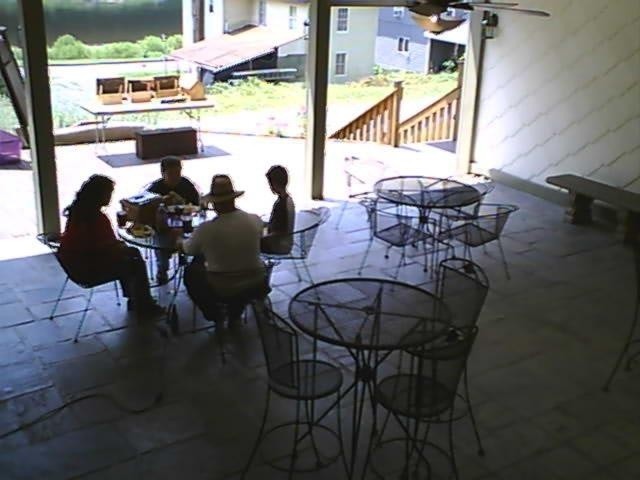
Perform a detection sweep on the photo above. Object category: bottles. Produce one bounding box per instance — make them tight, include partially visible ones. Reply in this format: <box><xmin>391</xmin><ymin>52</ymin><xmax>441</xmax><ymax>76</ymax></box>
<box><xmin>156</xmin><ymin>203</ymin><xmax>206</xmax><ymax>238</ymax></box>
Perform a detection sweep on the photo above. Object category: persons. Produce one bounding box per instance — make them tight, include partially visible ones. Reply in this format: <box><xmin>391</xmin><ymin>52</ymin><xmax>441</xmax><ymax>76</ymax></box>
<box><xmin>176</xmin><ymin>176</ymin><xmax>267</xmax><ymax>332</ymax></box>
<box><xmin>61</xmin><ymin>175</ymin><xmax>166</xmax><ymax>315</ymax></box>
<box><xmin>144</xmin><ymin>156</ymin><xmax>199</xmax><ymax>282</ymax></box>
<box><xmin>260</xmin><ymin>165</ymin><xmax>296</xmax><ymax>254</ymax></box>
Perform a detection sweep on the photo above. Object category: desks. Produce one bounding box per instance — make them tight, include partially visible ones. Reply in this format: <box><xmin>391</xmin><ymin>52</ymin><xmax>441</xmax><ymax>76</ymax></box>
<box><xmin>75</xmin><ymin>94</ymin><xmax>215</xmax><ymax>158</ymax></box>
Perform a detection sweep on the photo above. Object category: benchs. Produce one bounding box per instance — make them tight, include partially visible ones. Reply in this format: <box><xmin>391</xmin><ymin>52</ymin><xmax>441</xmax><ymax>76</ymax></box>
<box><xmin>133</xmin><ymin>125</ymin><xmax>199</xmax><ymax>161</ymax></box>
<box><xmin>544</xmin><ymin>171</ymin><xmax>639</xmax><ymax>245</ymax></box>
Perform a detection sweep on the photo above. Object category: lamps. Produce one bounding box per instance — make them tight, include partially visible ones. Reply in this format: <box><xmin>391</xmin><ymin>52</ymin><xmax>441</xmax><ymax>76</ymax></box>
<box><xmin>480</xmin><ymin>14</ymin><xmax>498</xmax><ymax>39</ymax></box>
<box><xmin>407</xmin><ymin>7</ymin><xmax>469</xmax><ymax>34</ymax></box>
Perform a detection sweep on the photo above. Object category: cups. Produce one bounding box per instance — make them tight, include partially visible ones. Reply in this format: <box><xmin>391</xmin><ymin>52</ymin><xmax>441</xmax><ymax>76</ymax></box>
<box><xmin>116</xmin><ymin>211</ymin><xmax>127</xmax><ymax>227</ymax></box>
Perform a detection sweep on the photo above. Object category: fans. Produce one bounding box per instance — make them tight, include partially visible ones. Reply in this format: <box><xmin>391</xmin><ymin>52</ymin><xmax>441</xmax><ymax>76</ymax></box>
<box><xmin>321</xmin><ymin>1</ymin><xmax>550</xmax><ymax>37</ymax></box>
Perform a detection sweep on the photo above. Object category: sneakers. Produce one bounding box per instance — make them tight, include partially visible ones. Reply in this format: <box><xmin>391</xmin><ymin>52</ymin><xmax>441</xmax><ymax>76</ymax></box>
<box><xmin>229</xmin><ymin>311</ymin><xmax>241</xmax><ymax>328</ymax></box>
<box><xmin>127</xmin><ymin>296</ymin><xmax>166</xmax><ymax>314</ymax></box>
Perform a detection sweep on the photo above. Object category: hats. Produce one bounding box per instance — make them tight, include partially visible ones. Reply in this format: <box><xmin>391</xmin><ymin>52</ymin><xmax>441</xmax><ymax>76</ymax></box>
<box><xmin>201</xmin><ymin>174</ymin><xmax>244</xmax><ymax>201</ymax></box>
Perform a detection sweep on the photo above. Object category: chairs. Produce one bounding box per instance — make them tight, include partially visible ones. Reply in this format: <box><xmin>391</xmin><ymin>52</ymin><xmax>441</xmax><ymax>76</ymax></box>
<box><xmin>182</xmin><ymin>262</ymin><xmax>276</xmax><ymax>364</ymax></box>
<box><xmin>117</xmin><ymin>167</ymin><xmax>331</xmax><ymax>323</ymax></box>
<box><xmin>360</xmin><ymin>171</ymin><xmax>517</xmax><ymax>282</ymax></box>
<box><xmin>35</xmin><ymin>232</ymin><xmax>121</xmax><ymax>343</ymax></box>
<box><xmin>245</xmin><ymin>254</ymin><xmax>492</xmax><ymax>478</ymax></box>
